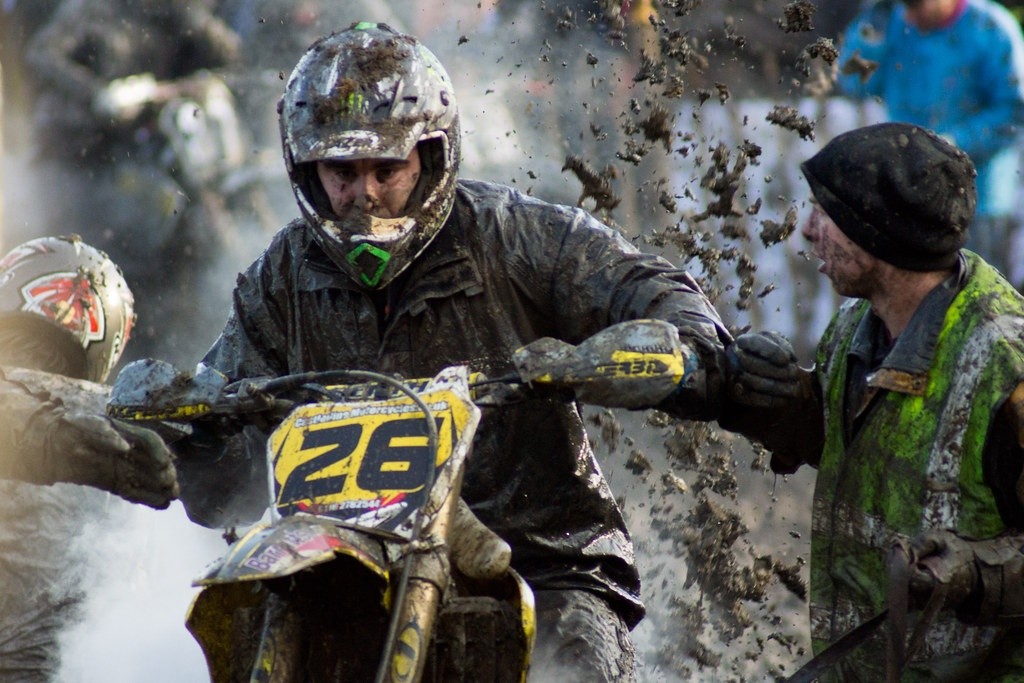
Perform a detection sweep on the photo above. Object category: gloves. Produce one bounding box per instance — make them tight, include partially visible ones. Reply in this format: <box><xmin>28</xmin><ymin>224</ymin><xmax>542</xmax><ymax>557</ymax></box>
<box><xmin>887</xmin><ymin>530</ymin><xmax>975</xmax><ymax>613</ymax></box>
<box><xmin>23</xmin><ymin>403</ymin><xmax>179</xmax><ymax>509</ymax></box>
<box><xmin>725</xmin><ymin>331</ymin><xmax>805</xmax><ymax>420</ymax></box>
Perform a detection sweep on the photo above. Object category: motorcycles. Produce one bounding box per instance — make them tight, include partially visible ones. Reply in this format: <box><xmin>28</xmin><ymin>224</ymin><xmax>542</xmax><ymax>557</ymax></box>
<box><xmin>105</xmin><ymin>316</ymin><xmax>694</xmax><ymax>682</ymax></box>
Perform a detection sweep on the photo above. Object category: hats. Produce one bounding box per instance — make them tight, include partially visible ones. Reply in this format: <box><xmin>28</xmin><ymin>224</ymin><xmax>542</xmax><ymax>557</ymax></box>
<box><xmin>799</xmin><ymin>122</ymin><xmax>977</xmax><ymax>272</ymax></box>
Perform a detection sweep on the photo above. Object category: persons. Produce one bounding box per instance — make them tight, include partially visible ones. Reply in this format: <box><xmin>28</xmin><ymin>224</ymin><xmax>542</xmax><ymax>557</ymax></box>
<box><xmin>0</xmin><ymin>365</ymin><xmax>179</xmax><ymax>511</ymax></box>
<box><xmin>724</xmin><ymin>120</ymin><xmax>1024</xmax><ymax>683</ymax></box>
<box><xmin>167</xmin><ymin>19</ymin><xmax>738</xmax><ymax>683</ymax></box>
<box><xmin>0</xmin><ymin>236</ymin><xmax>139</xmax><ymax>683</ymax></box>
<box><xmin>815</xmin><ymin>0</ymin><xmax>1024</xmax><ymax>298</ymax></box>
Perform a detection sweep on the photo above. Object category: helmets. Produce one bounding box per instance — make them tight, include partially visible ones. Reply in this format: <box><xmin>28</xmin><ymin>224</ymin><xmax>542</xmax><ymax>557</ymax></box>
<box><xmin>0</xmin><ymin>236</ymin><xmax>134</xmax><ymax>383</ymax></box>
<box><xmin>277</xmin><ymin>22</ymin><xmax>461</xmax><ymax>291</ymax></box>
